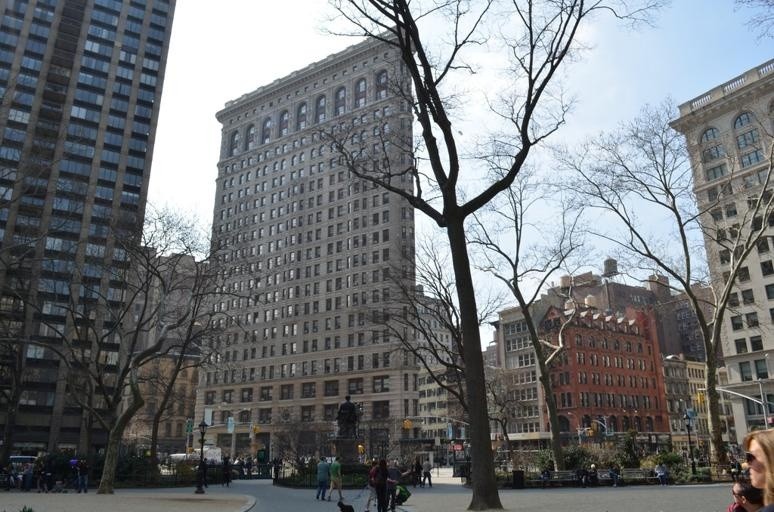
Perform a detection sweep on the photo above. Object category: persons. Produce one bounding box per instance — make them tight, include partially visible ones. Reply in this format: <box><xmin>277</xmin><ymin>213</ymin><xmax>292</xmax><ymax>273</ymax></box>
<box><xmin>301</xmin><ymin>455</ymin><xmax>305</xmax><ymax>463</ymax></box>
<box><xmin>2</xmin><ymin>457</ymin><xmax>92</xmax><ymax>493</ymax></box>
<box><xmin>334</xmin><ymin>395</ymin><xmax>358</xmax><ymax>438</ymax></box>
<box><xmin>577</xmin><ymin>464</ymin><xmax>589</xmax><ymax>488</ymax></box>
<box><xmin>608</xmin><ymin>462</ymin><xmax>620</xmax><ymax>487</ymax></box>
<box><xmin>588</xmin><ymin>464</ymin><xmax>599</xmax><ymax>488</ymax></box>
<box><xmin>199</xmin><ymin>457</ymin><xmax>209</xmax><ymax>488</ymax></box>
<box><xmin>316</xmin><ymin>454</ymin><xmax>432</xmax><ymax>512</ymax></box>
<box><xmin>654</xmin><ymin>460</ymin><xmax>667</xmax><ymax>487</ymax></box>
<box><xmin>221</xmin><ymin>454</ymin><xmax>285</xmax><ymax>487</ymax></box>
<box><xmin>540</xmin><ymin>467</ymin><xmax>552</xmax><ymax>487</ymax></box>
<box><xmin>309</xmin><ymin>455</ymin><xmax>317</xmax><ymax>464</ymax></box>
<box><xmin>727</xmin><ymin>428</ymin><xmax>774</xmax><ymax>512</ymax></box>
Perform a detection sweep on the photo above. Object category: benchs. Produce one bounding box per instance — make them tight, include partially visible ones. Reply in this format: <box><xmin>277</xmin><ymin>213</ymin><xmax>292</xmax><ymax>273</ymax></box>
<box><xmin>527</xmin><ymin>468</ymin><xmax>668</xmax><ymax>482</ymax></box>
<box><xmin>716</xmin><ymin>464</ymin><xmax>732</xmax><ymax>478</ymax></box>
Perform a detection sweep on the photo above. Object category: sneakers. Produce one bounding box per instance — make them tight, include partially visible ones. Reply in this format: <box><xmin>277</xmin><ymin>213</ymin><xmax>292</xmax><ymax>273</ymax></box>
<box><xmin>317</xmin><ymin>494</ymin><xmax>345</xmax><ymax>501</ymax></box>
<box><xmin>413</xmin><ymin>484</ymin><xmax>432</xmax><ymax>488</ymax></box>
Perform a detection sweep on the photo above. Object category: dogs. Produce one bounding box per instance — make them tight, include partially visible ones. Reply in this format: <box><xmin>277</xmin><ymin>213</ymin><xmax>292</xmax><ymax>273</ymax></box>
<box><xmin>337</xmin><ymin>501</ymin><xmax>354</xmax><ymax>512</ymax></box>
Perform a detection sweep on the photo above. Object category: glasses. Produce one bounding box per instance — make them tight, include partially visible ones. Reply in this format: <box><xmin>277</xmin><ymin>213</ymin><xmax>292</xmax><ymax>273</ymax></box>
<box><xmin>746</xmin><ymin>452</ymin><xmax>758</xmax><ymax>464</ymax></box>
<box><xmin>732</xmin><ymin>491</ymin><xmax>741</xmax><ymax>495</ymax></box>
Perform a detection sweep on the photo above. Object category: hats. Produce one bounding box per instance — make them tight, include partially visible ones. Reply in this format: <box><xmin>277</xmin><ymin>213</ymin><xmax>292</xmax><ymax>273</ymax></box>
<box><xmin>372</xmin><ymin>462</ymin><xmax>376</xmax><ymax>466</ymax></box>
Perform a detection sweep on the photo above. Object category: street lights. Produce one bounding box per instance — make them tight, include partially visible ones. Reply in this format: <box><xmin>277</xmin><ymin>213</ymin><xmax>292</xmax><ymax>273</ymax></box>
<box><xmin>450</xmin><ymin>439</ymin><xmax>456</xmax><ymax>474</ymax></box>
<box><xmin>463</xmin><ymin>440</ymin><xmax>472</xmax><ymax>486</ymax></box>
<box><xmin>697</xmin><ymin>388</ymin><xmax>769</xmax><ymax>430</ymax></box>
<box><xmin>568</xmin><ymin>412</ymin><xmax>582</xmax><ymax>447</ymax></box>
<box><xmin>592</xmin><ymin>420</ymin><xmax>608</xmax><ymax>436</ymax></box>
<box><xmin>683</xmin><ymin>413</ymin><xmax>697</xmax><ymax>475</ymax></box>
<box><xmin>195</xmin><ymin>421</ymin><xmax>208</xmax><ymax>494</ymax></box>
